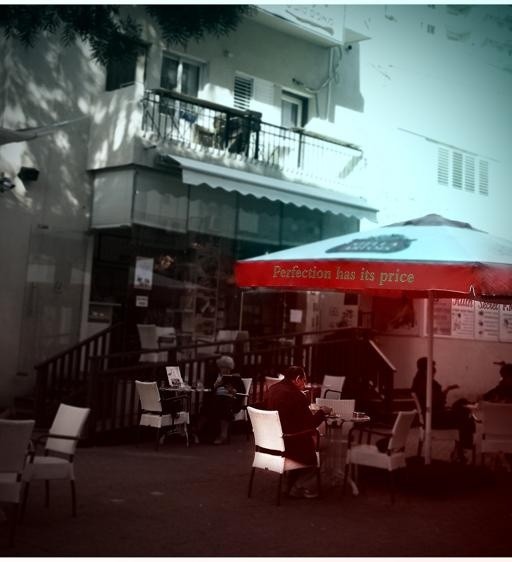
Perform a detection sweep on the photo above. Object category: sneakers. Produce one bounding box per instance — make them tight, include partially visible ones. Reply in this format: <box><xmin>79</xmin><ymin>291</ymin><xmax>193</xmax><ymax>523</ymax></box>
<box><xmin>288</xmin><ymin>486</ymin><xmax>304</xmax><ymax>499</ymax></box>
<box><xmin>303</xmin><ymin>488</ymin><xmax>319</xmax><ymax>498</ymax></box>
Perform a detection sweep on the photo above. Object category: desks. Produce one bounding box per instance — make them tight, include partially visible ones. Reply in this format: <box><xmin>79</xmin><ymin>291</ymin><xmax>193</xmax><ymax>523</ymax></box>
<box><xmin>160</xmin><ymin>103</ymin><xmax>198</xmax><ymax>141</ymax></box>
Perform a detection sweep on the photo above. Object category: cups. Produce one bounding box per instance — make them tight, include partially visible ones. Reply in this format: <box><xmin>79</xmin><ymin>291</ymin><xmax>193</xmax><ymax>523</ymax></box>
<box><xmin>310</xmin><ymin>403</ymin><xmax>320</xmax><ymax>410</ymax></box>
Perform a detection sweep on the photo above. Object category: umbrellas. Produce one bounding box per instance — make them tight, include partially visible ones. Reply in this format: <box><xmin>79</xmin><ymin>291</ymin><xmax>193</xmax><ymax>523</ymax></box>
<box><xmin>233</xmin><ymin>213</ymin><xmax>512</xmax><ymax>464</ymax></box>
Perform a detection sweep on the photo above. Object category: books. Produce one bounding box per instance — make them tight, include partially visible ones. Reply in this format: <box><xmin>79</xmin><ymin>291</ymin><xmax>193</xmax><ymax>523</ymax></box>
<box><xmin>218</xmin><ymin>373</ymin><xmax>241</xmax><ymax>387</ymax></box>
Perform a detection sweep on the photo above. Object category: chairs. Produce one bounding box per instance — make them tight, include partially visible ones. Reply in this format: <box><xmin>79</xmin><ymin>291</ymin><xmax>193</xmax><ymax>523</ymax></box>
<box><xmin>194</xmin><ymin>115</ymin><xmax>219</xmax><ymax>148</ymax></box>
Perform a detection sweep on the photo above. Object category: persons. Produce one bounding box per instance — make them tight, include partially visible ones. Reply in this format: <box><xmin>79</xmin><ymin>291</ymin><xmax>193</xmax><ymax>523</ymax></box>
<box><xmin>413</xmin><ymin>356</ymin><xmax>477</xmax><ymax>458</ymax></box>
<box><xmin>453</xmin><ymin>363</ymin><xmax>512</xmax><ymax>410</ymax></box>
<box><xmin>262</xmin><ymin>365</ymin><xmax>333</xmax><ymax>500</ymax></box>
<box><xmin>191</xmin><ymin>355</ymin><xmax>246</xmax><ymax>444</ymax></box>
